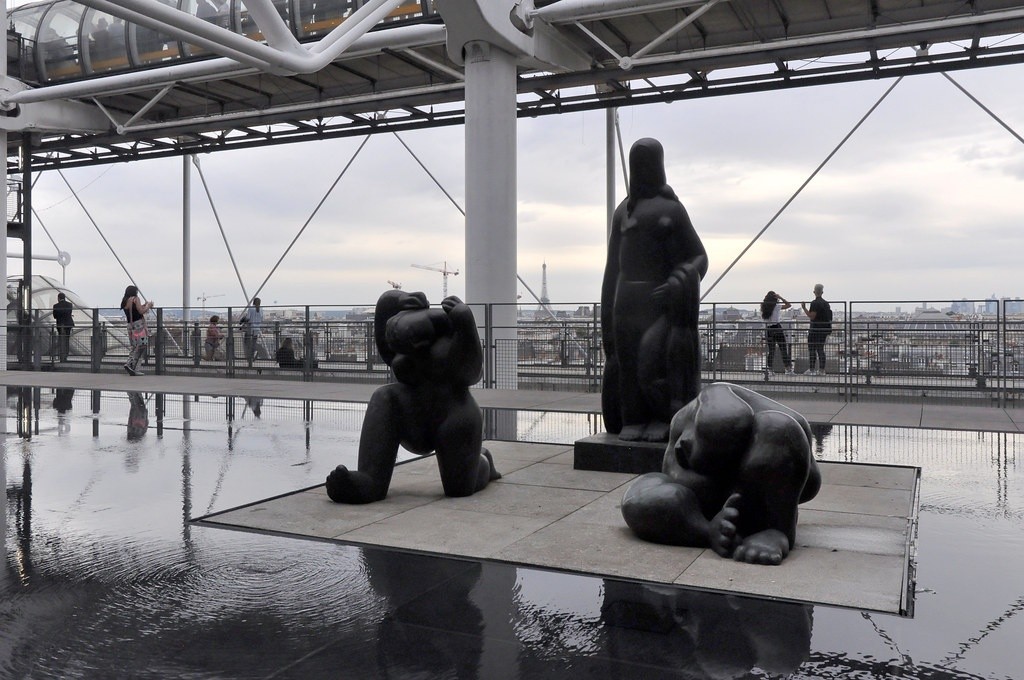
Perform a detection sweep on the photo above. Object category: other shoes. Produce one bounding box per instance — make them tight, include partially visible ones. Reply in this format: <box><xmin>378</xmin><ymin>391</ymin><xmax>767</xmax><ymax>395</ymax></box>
<box><xmin>135</xmin><ymin>369</ymin><xmax>144</xmax><ymax>375</ymax></box>
<box><xmin>124</xmin><ymin>365</ymin><xmax>138</xmax><ymax>375</ymax></box>
<box><xmin>784</xmin><ymin>370</ymin><xmax>797</xmax><ymax>376</ymax></box>
<box><xmin>803</xmin><ymin>368</ymin><xmax>816</xmax><ymax>375</ymax></box>
<box><xmin>768</xmin><ymin>370</ymin><xmax>775</xmax><ymax>376</ymax></box>
<box><xmin>816</xmin><ymin>369</ymin><xmax>826</xmax><ymax>375</ymax></box>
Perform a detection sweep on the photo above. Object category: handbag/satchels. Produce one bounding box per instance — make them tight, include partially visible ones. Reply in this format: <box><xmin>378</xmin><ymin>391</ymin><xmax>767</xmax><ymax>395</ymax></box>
<box><xmin>130</xmin><ymin>329</ymin><xmax>148</xmax><ymax>347</ymax></box>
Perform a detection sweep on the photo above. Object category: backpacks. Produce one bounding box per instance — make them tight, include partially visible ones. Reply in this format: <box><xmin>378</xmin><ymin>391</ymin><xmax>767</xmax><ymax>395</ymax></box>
<box><xmin>817</xmin><ymin>308</ymin><xmax>833</xmax><ymax>335</ymax></box>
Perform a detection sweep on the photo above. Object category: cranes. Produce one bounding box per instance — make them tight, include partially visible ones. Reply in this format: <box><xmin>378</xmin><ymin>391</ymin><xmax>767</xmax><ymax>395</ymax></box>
<box><xmin>410</xmin><ymin>260</ymin><xmax>460</xmax><ymax>301</ymax></box>
<box><xmin>197</xmin><ymin>292</ymin><xmax>226</xmax><ymax>319</ymax></box>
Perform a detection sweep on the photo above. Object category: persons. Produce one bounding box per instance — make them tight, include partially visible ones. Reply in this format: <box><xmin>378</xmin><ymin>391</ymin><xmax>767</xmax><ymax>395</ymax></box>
<box><xmin>201</xmin><ymin>315</ymin><xmax>223</xmax><ymax>362</ymax></box>
<box><xmin>276</xmin><ymin>338</ymin><xmax>319</xmax><ymax>368</ymax></box>
<box><xmin>47</xmin><ymin>0</ymin><xmax>417</xmax><ymax>65</ymax></box>
<box><xmin>801</xmin><ymin>283</ymin><xmax>833</xmax><ymax>376</ymax></box>
<box><xmin>759</xmin><ymin>291</ymin><xmax>797</xmax><ymax>376</ymax></box>
<box><xmin>239</xmin><ymin>298</ymin><xmax>265</xmax><ymax>367</ymax></box>
<box><xmin>52</xmin><ymin>292</ymin><xmax>75</xmax><ymax>364</ymax></box>
<box><xmin>594</xmin><ymin>135</ymin><xmax>707</xmax><ymax>443</ymax></box>
<box><xmin>120</xmin><ymin>284</ymin><xmax>154</xmax><ymax>376</ymax></box>
